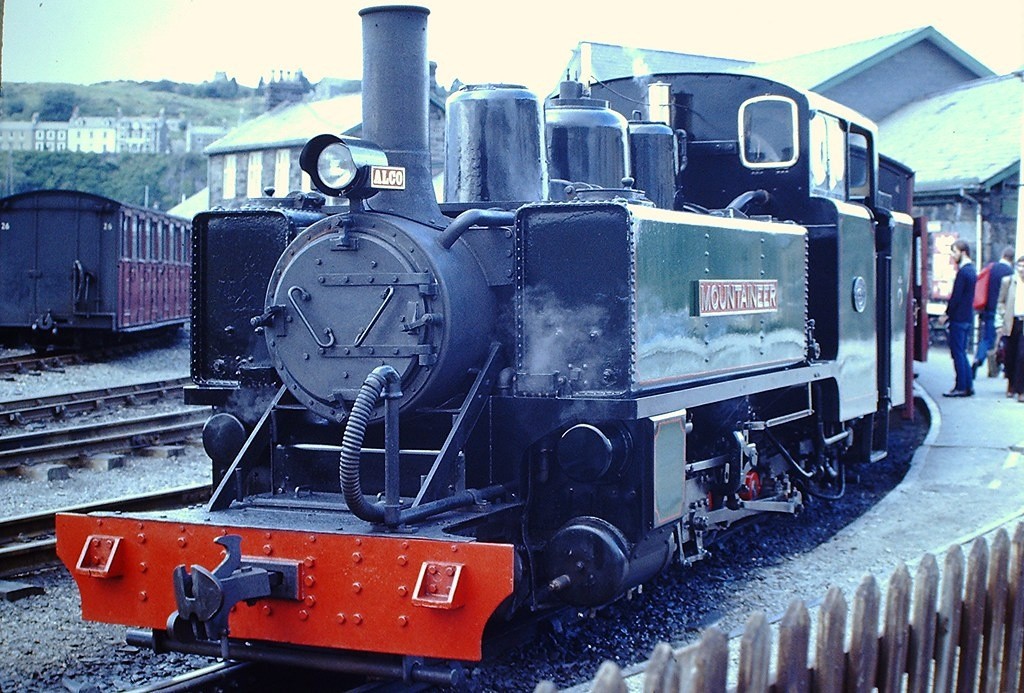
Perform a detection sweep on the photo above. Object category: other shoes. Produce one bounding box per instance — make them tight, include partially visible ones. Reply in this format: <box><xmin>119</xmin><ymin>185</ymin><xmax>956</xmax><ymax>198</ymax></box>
<box><xmin>943</xmin><ymin>388</ymin><xmax>973</xmax><ymax>397</ymax></box>
<box><xmin>1007</xmin><ymin>390</ymin><xmax>1013</xmax><ymax>397</ymax></box>
<box><xmin>972</xmin><ymin>361</ymin><xmax>977</xmax><ymax>372</ymax></box>
<box><xmin>1019</xmin><ymin>392</ymin><xmax>1024</xmax><ymax>401</ymax></box>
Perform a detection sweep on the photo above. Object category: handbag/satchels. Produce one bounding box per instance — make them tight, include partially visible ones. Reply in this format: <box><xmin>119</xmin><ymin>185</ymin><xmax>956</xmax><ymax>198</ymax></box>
<box><xmin>973</xmin><ymin>262</ymin><xmax>994</xmax><ymax>309</ymax></box>
<box><xmin>986</xmin><ymin>332</ymin><xmax>1000</xmax><ymax>377</ymax></box>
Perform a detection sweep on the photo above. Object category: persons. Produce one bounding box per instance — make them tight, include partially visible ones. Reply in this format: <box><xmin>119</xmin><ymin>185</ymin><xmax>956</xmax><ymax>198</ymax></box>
<box><xmin>939</xmin><ymin>241</ymin><xmax>977</xmax><ymax>398</ymax></box>
<box><xmin>972</xmin><ymin>245</ymin><xmax>1024</xmax><ymax>403</ymax></box>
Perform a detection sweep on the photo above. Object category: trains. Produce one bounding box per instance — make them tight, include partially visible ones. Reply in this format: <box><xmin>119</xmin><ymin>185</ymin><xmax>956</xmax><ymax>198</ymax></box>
<box><xmin>0</xmin><ymin>189</ymin><xmax>193</xmax><ymax>348</ymax></box>
<box><xmin>54</xmin><ymin>0</ymin><xmax>935</xmax><ymax>686</ymax></box>
